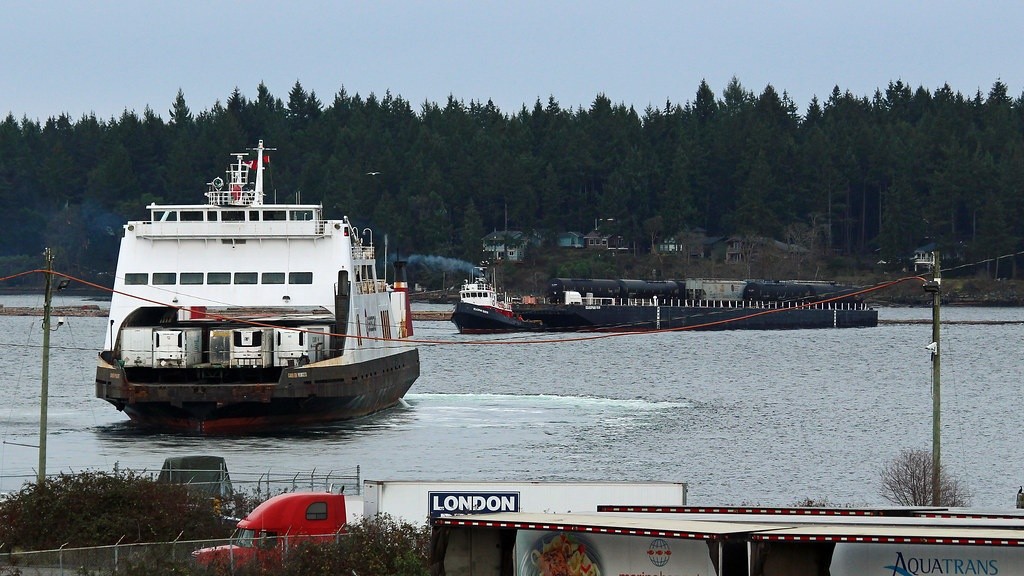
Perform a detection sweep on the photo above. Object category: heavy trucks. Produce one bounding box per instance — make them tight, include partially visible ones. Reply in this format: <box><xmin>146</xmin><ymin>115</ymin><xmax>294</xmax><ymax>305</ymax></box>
<box><xmin>189</xmin><ymin>479</ymin><xmax>693</xmax><ymax>575</ymax></box>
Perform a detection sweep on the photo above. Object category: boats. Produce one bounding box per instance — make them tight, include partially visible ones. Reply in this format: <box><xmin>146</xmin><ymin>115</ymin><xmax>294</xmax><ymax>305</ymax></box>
<box><xmin>447</xmin><ymin>259</ymin><xmax>879</xmax><ymax>332</ymax></box>
<box><xmin>87</xmin><ymin>137</ymin><xmax>422</xmax><ymax>432</ymax></box>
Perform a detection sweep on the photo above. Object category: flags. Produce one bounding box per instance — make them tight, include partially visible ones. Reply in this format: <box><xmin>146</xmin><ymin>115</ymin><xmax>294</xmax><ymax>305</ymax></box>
<box><xmin>243</xmin><ymin>154</ymin><xmax>271</xmax><ymax>173</ymax></box>
